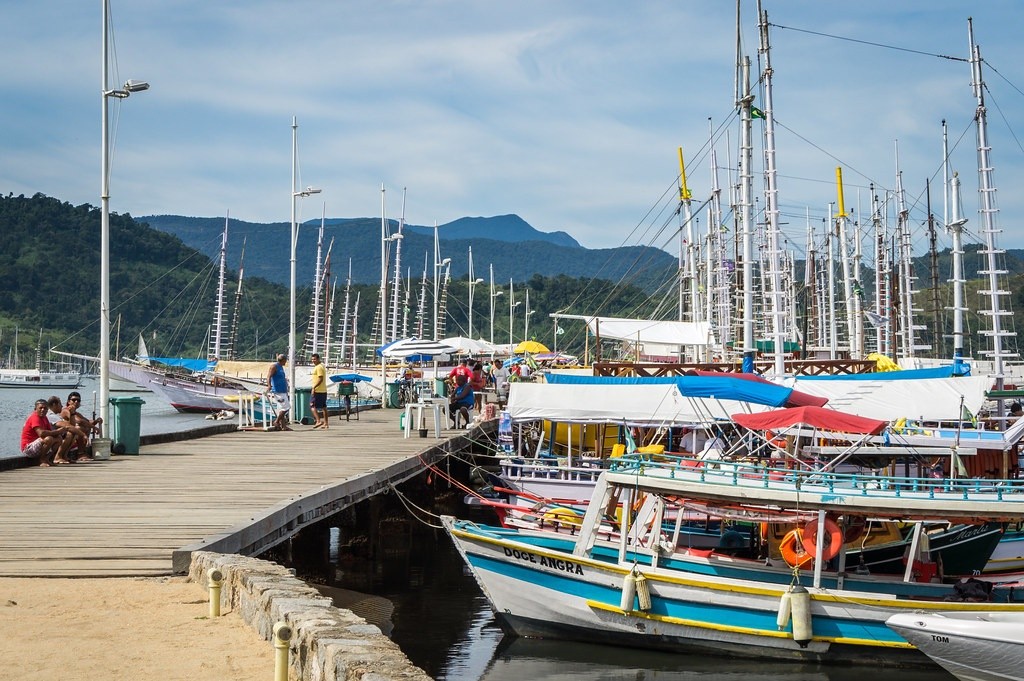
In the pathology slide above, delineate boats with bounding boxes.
[880,608,1024,681]
[400,337,1024,657]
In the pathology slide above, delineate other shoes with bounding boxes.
[75,453,94,461]
[461,425,466,429]
[451,422,460,429]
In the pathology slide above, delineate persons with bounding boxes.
[678,429,727,454]
[512,361,530,377]
[400,363,415,387]
[449,358,510,414]
[442,377,474,428]
[927,463,956,492]
[310,353,328,428]
[539,360,547,369]
[267,355,293,431]
[20,392,103,467]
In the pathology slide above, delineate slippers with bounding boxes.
[39,460,50,467]
[273,422,281,431]
[53,459,70,464]
[282,427,293,431]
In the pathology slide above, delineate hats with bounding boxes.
[1011,403,1022,412]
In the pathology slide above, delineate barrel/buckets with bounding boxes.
[91,438,112,461]
[419,429,428,437]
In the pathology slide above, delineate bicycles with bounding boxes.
[390,375,418,413]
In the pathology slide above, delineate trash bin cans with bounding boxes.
[386,381,404,408]
[109,397,146,455]
[295,387,315,425]
[435,377,449,398]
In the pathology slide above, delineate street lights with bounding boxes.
[284,115,324,423]
[524,289,536,342]
[85,0,147,461]
[488,262,504,342]
[468,245,484,339]
[433,219,452,344]
[509,277,522,348]
[379,181,404,413]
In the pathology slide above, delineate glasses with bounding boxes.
[70,398,81,404]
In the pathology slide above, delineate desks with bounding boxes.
[403,404,441,440]
[417,397,449,430]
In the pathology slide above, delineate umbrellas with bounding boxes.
[503,357,524,366]
[531,354,568,361]
[514,339,549,353]
[378,335,459,427]
[440,337,508,356]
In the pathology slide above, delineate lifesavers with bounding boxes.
[781,511,867,567]
